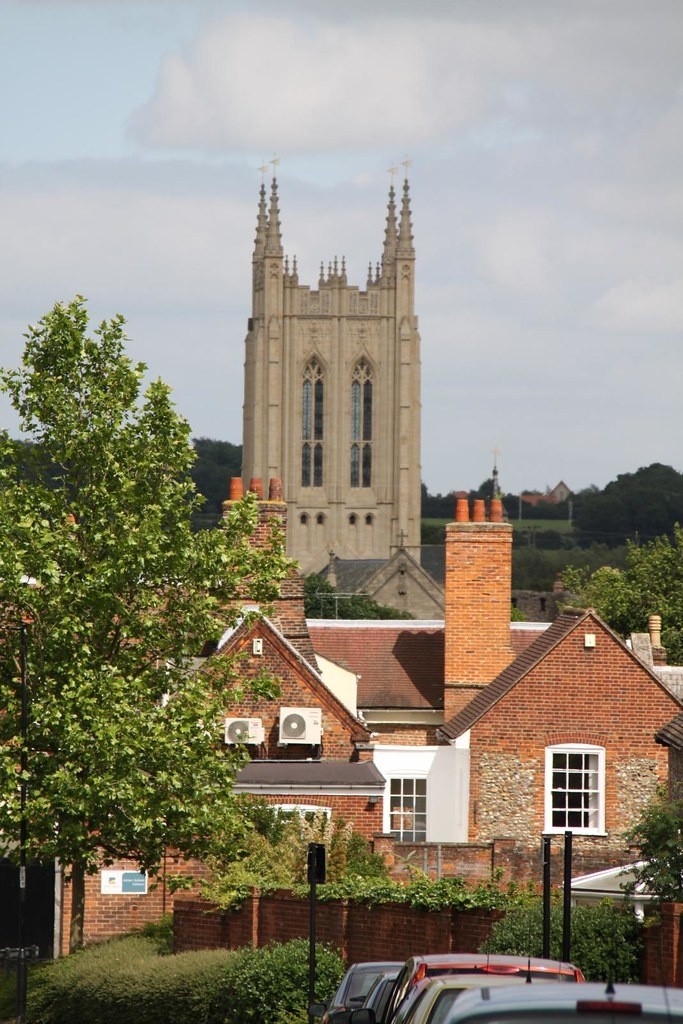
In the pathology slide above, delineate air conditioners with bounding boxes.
[279,706,321,745]
[224,718,262,745]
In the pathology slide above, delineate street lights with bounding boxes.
[17,576,37,1023]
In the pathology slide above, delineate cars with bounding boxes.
[307,951,683,1024]
